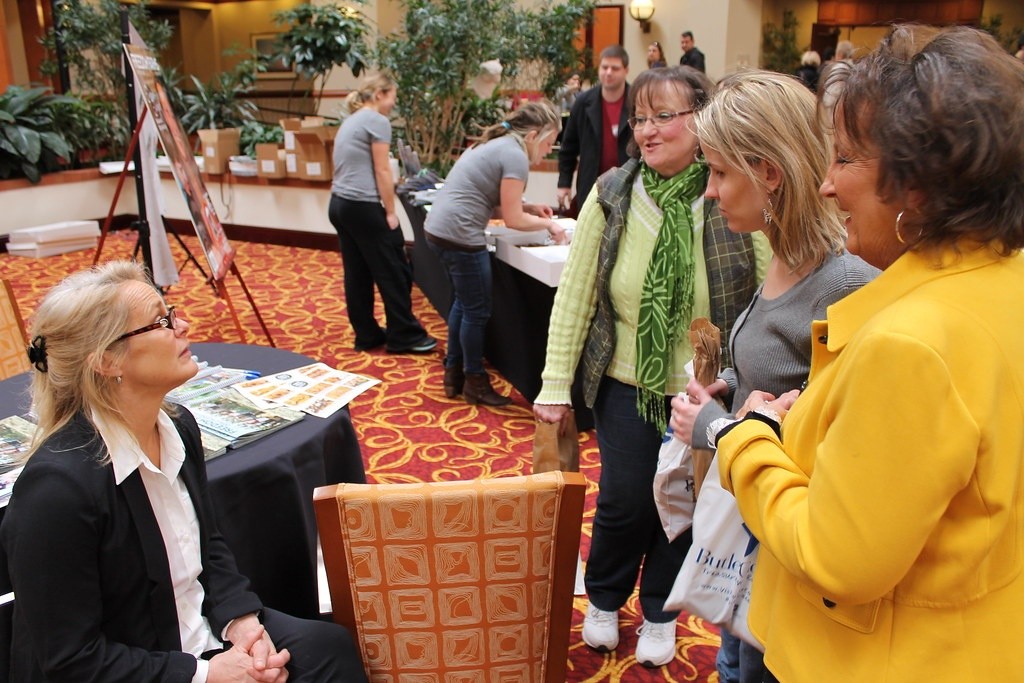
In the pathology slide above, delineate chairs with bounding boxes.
[316,472,587,683]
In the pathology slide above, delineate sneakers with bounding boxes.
[410,337,438,351]
[581,601,619,652]
[635,614,677,667]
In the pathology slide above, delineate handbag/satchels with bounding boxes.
[533,409,579,474]
[653,391,697,544]
[662,449,769,654]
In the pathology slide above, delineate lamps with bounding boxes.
[629,0,655,32]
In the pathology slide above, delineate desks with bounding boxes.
[0,344,365,618]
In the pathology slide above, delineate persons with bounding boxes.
[551,31,705,219]
[536,66,774,665]
[423,97,568,402]
[703,25,1023,683]
[328,75,439,354]
[669,68,883,683]
[154,75,213,254]
[0,258,367,683]
[795,41,855,93]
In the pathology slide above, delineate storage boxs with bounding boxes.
[196,116,337,181]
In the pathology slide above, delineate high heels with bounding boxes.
[463,375,512,406]
[443,373,463,398]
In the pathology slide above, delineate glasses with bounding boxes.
[105,306,176,350]
[627,110,694,131]
